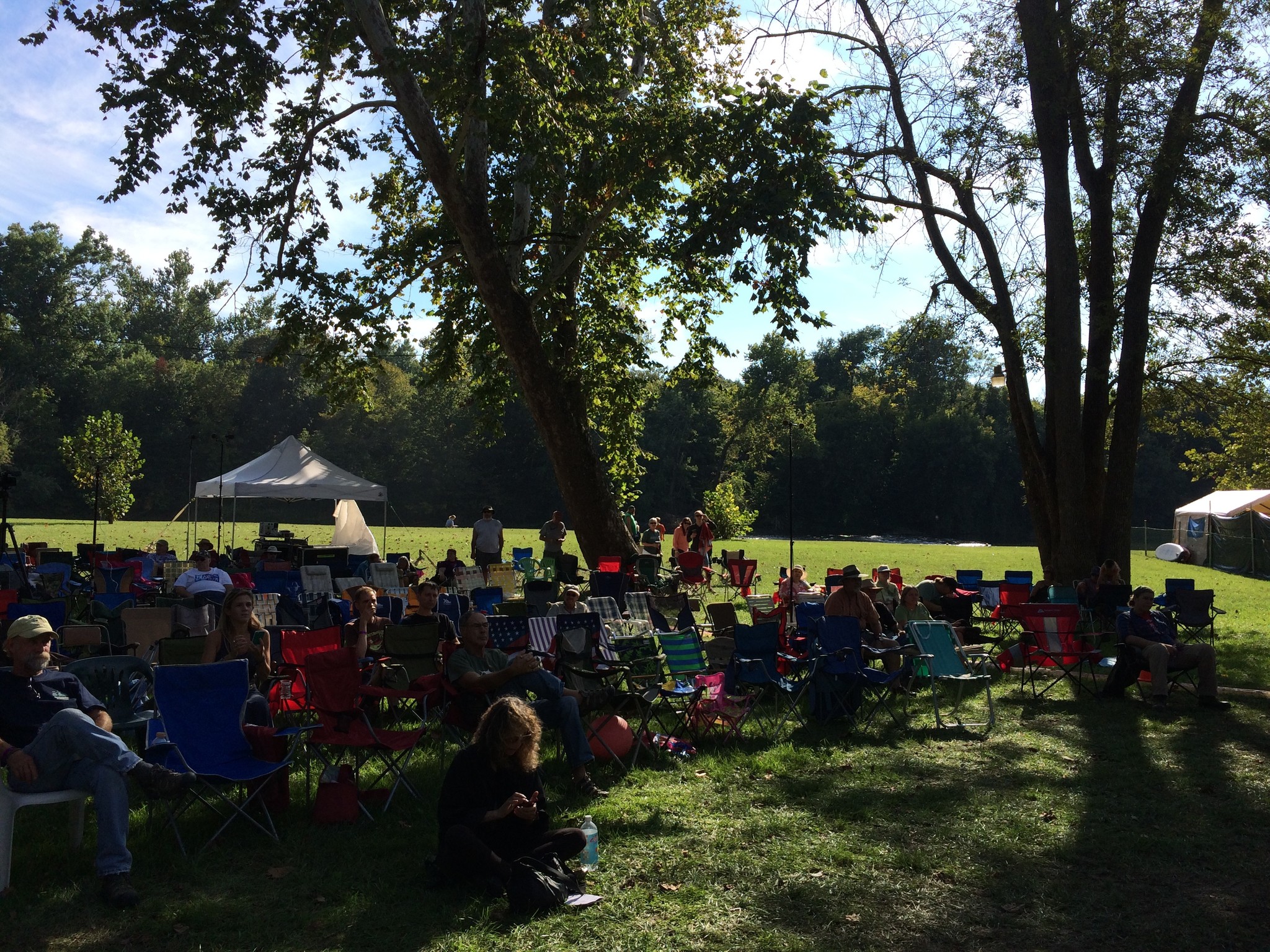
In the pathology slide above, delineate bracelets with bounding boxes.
[7,748,22,759]
[3,746,16,760]
[359,631,366,635]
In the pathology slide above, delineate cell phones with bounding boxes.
[253,631,265,645]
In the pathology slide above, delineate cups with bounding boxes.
[280,680,292,699]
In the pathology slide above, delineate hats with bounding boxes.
[786,565,807,580]
[263,546,282,554]
[682,517,692,525]
[1090,566,1100,574]
[7,614,60,641]
[196,550,211,558]
[196,539,213,550]
[944,577,958,592]
[877,565,892,574]
[155,540,168,546]
[860,579,884,594]
[480,505,495,514]
[564,584,580,597]
[838,565,867,585]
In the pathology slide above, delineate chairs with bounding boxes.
[0,540,1227,889]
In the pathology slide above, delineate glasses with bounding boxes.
[447,552,455,555]
[1092,574,1099,577]
[650,522,657,524]
[567,593,576,597]
[684,522,690,525]
[695,515,702,517]
[484,510,490,514]
[460,622,490,630]
[196,559,205,562]
[1043,570,1052,574]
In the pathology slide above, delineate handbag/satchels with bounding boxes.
[507,853,586,911]
[632,531,641,545]
[312,765,359,822]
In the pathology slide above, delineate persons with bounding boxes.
[255,546,285,572]
[624,505,717,595]
[470,505,504,585]
[781,559,1125,698]
[145,539,176,575]
[445,515,459,528]
[1116,586,1230,711]
[447,610,616,799]
[396,555,417,587]
[173,550,234,608]
[0,614,197,909]
[200,587,274,727]
[436,695,586,891]
[188,538,213,561]
[344,581,461,723]
[356,553,379,582]
[546,584,591,618]
[539,510,567,581]
[438,548,466,586]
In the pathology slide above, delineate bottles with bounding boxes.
[580,815,599,872]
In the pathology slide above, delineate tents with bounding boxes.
[1172,490,1270,566]
[194,435,387,561]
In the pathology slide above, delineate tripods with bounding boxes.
[0,488,33,600]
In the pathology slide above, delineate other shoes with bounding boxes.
[965,626,981,634]
[102,873,139,907]
[896,686,917,697]
[1153,696,1167,712]
[567,772,610,798]
[576,686,616,718]
[1198,695,1231,710]
[708,588,718,596]
[138,762,196,800]
